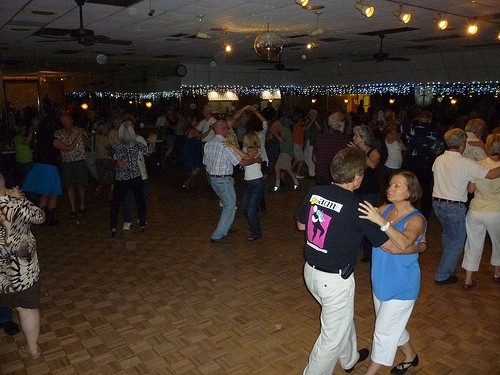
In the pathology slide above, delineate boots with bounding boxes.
[38,205,60,227]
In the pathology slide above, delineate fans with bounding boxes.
[351,35,411,62]
[257,53,301,71]
[36,0,132,46]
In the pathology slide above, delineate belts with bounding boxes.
[308,261,345,274]
[210,175,232,177]
[432,196,464,204]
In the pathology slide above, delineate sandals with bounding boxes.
[20,343,43,360]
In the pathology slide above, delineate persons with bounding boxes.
[295,148,426,375]
[0,88,500,242]
[459,133,499,290]
[0,186,32,334]
[355,170,426,375]
[429,127,500,285]
[231,132,267,241]
[0,171,47,357]
[202,117,263,245]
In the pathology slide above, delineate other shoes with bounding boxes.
[434,275,458,284]
[344,348,369,373]
[247,225,262,241]
[290,184,302,192]
[0,320,21,336]
[110,227,118,239]
[218,200,238,210]
[68,205,87,220]
[361,257,371,261]
[295,175,305,179]
[309,175,317,179]
[181,182,198,192]
[210,226,239,243]
[268,186,281,192]
[492,275,500,282]
[137,218,147,232]
[390,353,419,375]
[122,221,133,232]
[462,280,477,291]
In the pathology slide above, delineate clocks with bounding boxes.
[176,64,187,77]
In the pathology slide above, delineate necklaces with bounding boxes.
[389,205,413,224]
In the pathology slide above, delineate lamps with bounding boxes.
[432,10,448,30]
[355,1,376,18]
[307,36,320,49]
[220,31,232,52]
[295,0,310,7]
[392,3,411,24]
[465,17,479,36]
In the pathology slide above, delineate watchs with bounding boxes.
[381,222,390,231]
